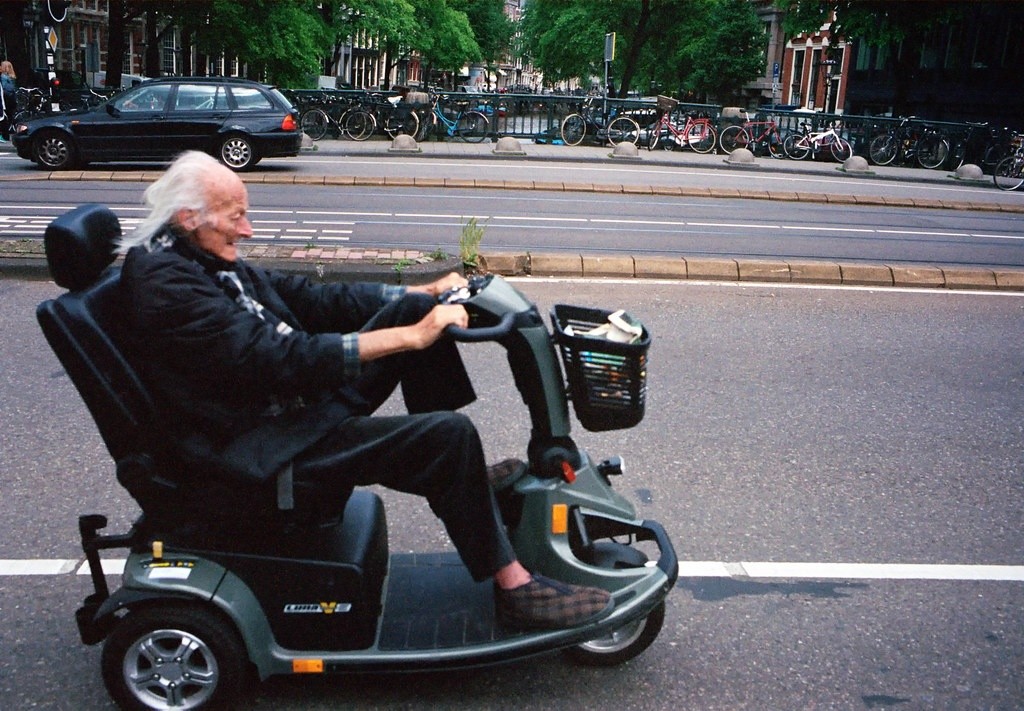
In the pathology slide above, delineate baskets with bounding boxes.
[550,304,652,432]
[658,95,678,112]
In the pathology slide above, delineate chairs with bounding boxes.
[35,201,388,603]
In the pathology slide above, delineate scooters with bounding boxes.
[34,206,681,711]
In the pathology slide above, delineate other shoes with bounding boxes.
[494,573,615,628]
[0,135,7,143]
[485,458,526,491]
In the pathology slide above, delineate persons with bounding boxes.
[116,152,615,630]
[124,90,166,111]
[474,72,482,92]
[0,61,17,143]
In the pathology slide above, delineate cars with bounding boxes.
[11,76,303,171]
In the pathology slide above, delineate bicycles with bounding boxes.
[9,81,1024,190]
